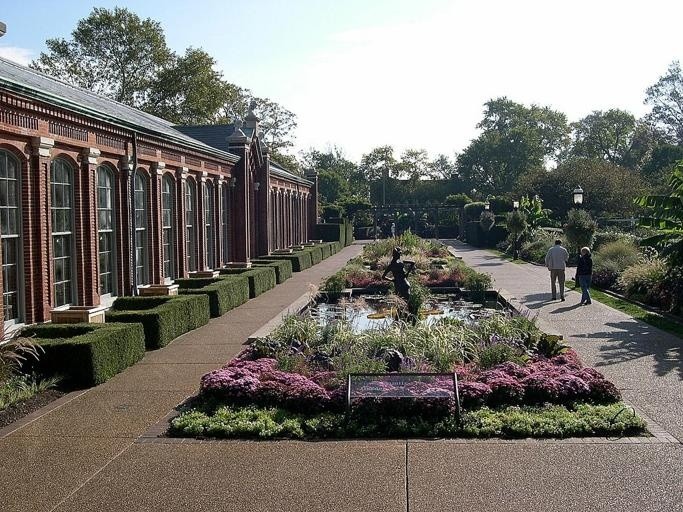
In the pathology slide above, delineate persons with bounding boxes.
[545,239,569,301]
[576,246,593,305]
[381,246,416,297]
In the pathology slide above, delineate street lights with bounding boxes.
[511,194,520,260]
[571,185,584,267]
[483,200,490,249]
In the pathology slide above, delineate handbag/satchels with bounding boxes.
[548,256,553,270]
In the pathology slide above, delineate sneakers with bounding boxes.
[586,301,591,304]
[552,298,556,300]
[562,299,565,301]
